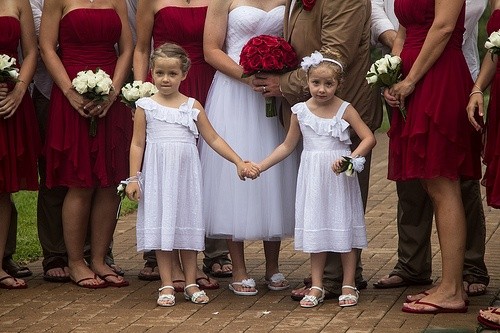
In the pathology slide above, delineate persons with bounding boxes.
[253,0,384,303]
[132,0,299,296]
[0,0,135,290]
[370,0,490,316]
[241,48,378,308]
[125,44,260,306]
[467,0,500,333]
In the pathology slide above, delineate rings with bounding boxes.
[14,103,16,107]
[97,105,102,109]
[263,87,266,92]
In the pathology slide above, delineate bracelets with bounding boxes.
[114,172,144,219]
[469,86,484,97]
[336,155,366,179]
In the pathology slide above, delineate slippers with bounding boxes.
[197,276,219,290]
[88,264,129,287]
[464,275,486,296]
[203,257,232,276]
[266,273,289,291]
[229,277,258,295]
[172,280,185,292]
[0,275,26,289]
[373,271,433,287]
[292,283,334,301]
[44,267,72,283]
[70,272,108,288]
[139,264,159,279]
[478,308,500,329]
[405,289,470,304]
[402,299,469,313]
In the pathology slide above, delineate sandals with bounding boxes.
[301,286,325,308]
[184,284,210,304]
[157,286,175,307]
[339,286,359,307]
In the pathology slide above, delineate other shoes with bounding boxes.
[2,257,31,276]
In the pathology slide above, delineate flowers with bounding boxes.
[119,80,158,109]
[365,53,407,119]
[484,28,500,62]
[70,68,115,135]
[0,53,25,85]
[239,34,300,117]
